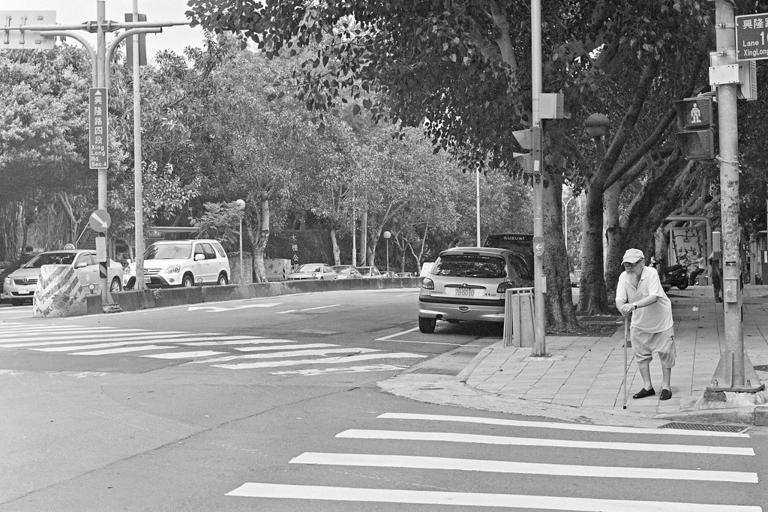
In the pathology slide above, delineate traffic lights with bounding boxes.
[509,129,533,173]
[671,97,714,161]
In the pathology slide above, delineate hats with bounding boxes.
[621,248,644,266]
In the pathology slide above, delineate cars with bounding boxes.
[418,233,534,333]
[287,263,413,279]
[0,239,231,305]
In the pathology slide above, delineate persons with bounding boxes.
[690,252,705,286]
[23,245,35,263]
[615,248,677,399]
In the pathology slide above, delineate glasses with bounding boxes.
[624,262,638,267]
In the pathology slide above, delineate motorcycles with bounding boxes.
[650,256,688,290]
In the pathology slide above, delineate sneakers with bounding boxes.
[660,389,672,400]
[633,387,655,399]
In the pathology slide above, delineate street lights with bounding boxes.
[383,231,391,276]
[237,199,245,275]
